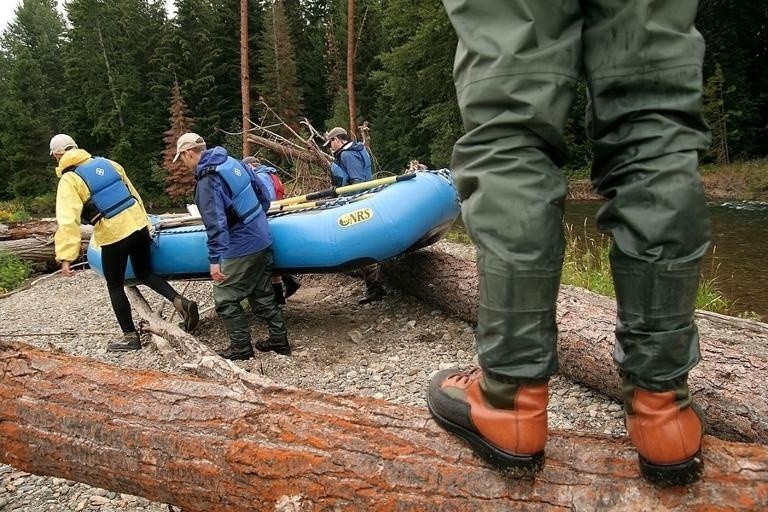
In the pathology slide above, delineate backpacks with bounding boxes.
[269,172,284,200]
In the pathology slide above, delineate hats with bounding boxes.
[323,127,347,147]
[172,133,206,163]
[242,156,258,164]
[49,134,78,156]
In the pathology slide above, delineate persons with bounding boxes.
[49,134,199,352]
[172,132,291,360]
[323,126,387,304]
[427,0,713,486]
[242,156,301,304]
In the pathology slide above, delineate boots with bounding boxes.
[272,282,286,305]
[282,273,299,298]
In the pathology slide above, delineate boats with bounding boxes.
[86,171,460,279]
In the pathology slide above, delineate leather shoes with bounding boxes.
[359,298,382,304]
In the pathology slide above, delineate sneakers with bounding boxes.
[621,381,705,485]
[427,370,548,475]
[174,296,199,330]
[255,335,291,355]
[108,332,142,352]
[215,342,254,360]
